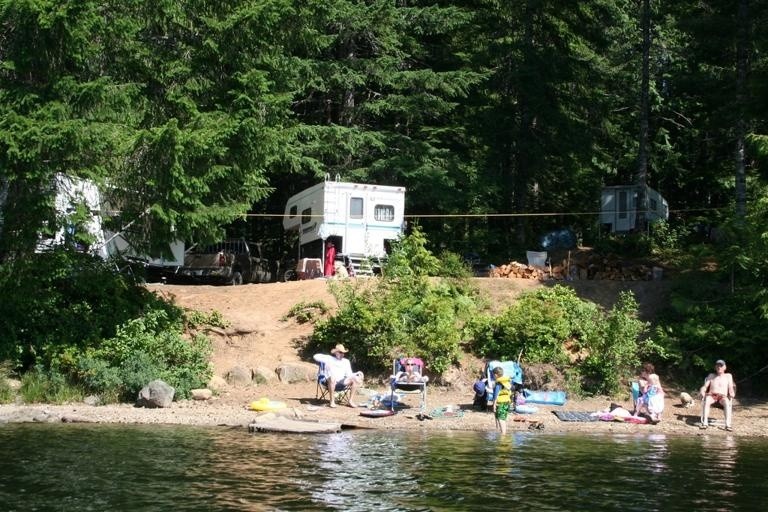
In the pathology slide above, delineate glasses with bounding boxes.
[407,363,413,366]
[338,351,345,354]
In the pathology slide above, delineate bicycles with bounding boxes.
[284,269,296,283]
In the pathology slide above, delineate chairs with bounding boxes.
[317,351,354,405]
[389,356,427,413]
[700,379,735,429]
[486,359,522,413]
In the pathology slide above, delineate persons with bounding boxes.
[396,359,429,382]
[636,360,665,419]
[323,343,363,408]
[492,367,512,435]
[700,360,736,430]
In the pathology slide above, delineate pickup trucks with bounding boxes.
[185,237,279,285]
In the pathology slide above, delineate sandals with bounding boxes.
[725,425,733,432]
[700,423,708,429]
[514,416,541,422]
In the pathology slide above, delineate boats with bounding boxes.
[248,413,342,433]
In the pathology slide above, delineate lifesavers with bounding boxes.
[251,397,286,410]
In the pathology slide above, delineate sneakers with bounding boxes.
[527,423,545,431]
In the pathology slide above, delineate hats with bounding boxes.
[473,381,485,397]
[715,360,725,366]
[331,343,349,354]
